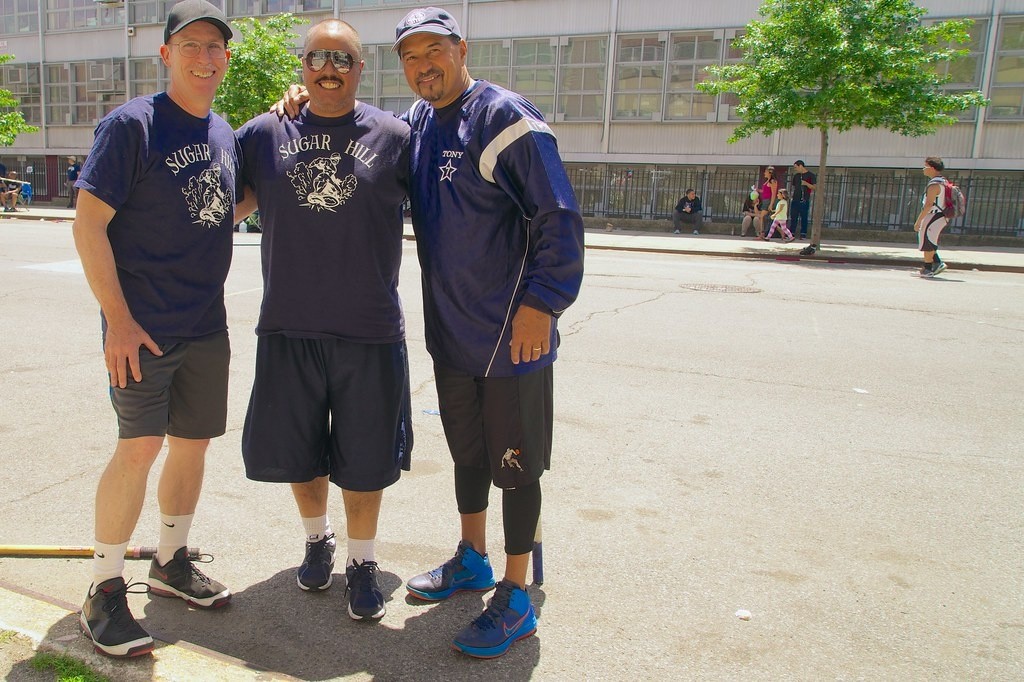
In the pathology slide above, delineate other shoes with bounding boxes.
[674,230,680,233]
[693,231,698,235]
[67,204,73,208]
[786,237,795,242]
[800,233,807,239]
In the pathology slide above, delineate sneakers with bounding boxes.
[406,540,497,601]
[451,578,536,659]
[78,577,155,659]
[147,547,232,609]
[296,532,336,590]
[933,263,947,275]
[344,557,384,620]
[911,267,934,277]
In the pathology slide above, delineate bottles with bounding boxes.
[239,221,248,233]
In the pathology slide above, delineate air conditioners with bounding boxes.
[8,68,37,84]
[90,64,120,81]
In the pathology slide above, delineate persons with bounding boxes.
[754,167,787,240]
[741,195,764,237]
[65,156,81,208]
[764,189,795,243]
[268,6,585,659]
[910,156,951,278]
[790,160,816,240]
[673,189,704,235]
[0,162,7,192]
[0,171,22,212]
[236,19,413,622]
[71,0,275,659]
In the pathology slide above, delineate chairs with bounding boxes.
[6,183,33,213]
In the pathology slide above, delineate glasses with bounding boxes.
[302,49,359,74]
[169,41,230,59]
[922,166,933,169]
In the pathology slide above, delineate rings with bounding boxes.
[534,347,541,351]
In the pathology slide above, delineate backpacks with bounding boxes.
[925,182,966,218]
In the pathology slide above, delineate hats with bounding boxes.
[390,7,462,53]
[750,191,759,200]
[67,156,76,162]
[164,0,233,45]
